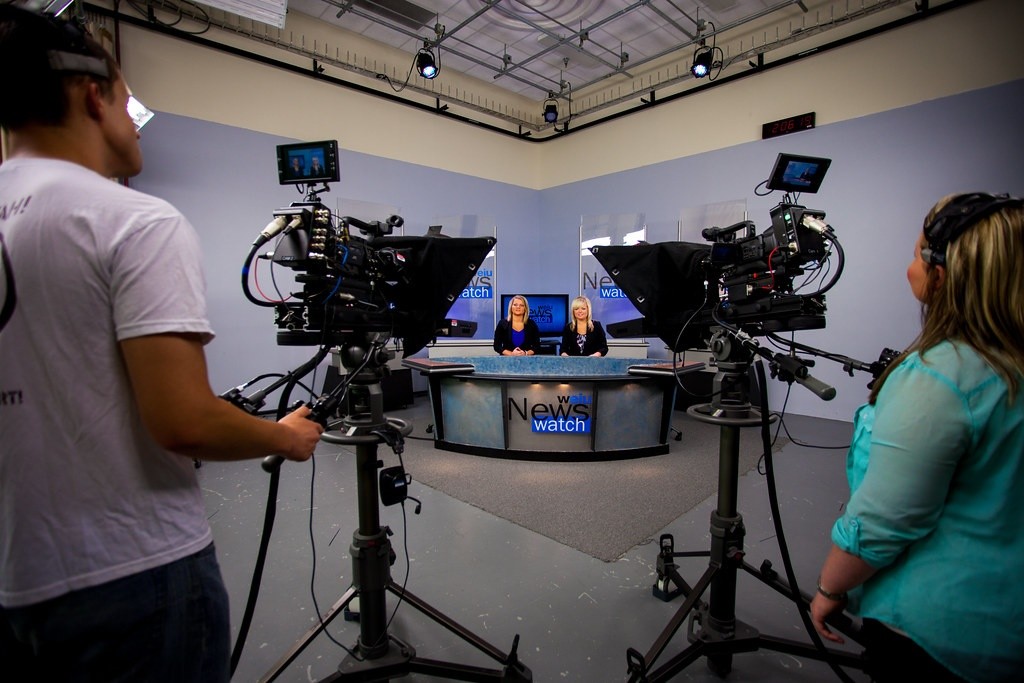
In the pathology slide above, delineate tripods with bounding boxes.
[622,326,873,683]
[217,327,532,683]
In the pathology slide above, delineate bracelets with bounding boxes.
[524,350,528,356]
[817,574,845,602]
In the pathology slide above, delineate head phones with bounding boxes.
[921,192,1024,267]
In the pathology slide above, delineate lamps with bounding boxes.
[690,45,714,78]
[542,98,560,122]
[414,47,438,79]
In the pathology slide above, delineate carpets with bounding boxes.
[321,392,794,562]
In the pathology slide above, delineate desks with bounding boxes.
[427,339,501,356]
[424,356,680,462]
[328,346,413,410]
[604,339,651,359]
[664,344,720,412]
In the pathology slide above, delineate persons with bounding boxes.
[309,157,324,176]
[559,296,608,357]
[290,158,304,178]
[493,295,540,356]
[0,0,326,683]
[808,193,1024,683]
[799,167,810,182]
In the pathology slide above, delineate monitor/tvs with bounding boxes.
[501,293,569,337]
